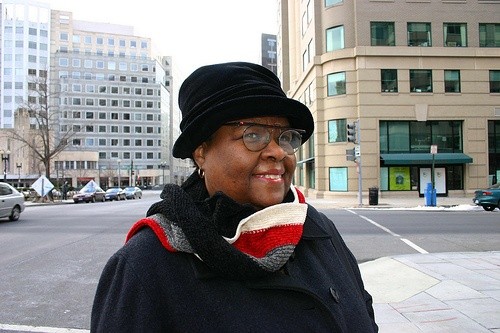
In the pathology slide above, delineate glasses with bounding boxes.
[222,121,306,156]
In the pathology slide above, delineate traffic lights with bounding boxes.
[132,170,135,175]
[346,121,356,143]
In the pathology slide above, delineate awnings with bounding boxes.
[380,152,473,164]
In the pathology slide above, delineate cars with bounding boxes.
[0,182,24,222]
[123,186,143,199]
[473,183,500,211]
[72,186,107,203]
[105,188,128,201]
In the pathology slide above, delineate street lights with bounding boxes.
[0,147,12,183]
[117,159,122,189]
[160,161,168,188]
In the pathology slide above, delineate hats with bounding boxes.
[172,62,315,160]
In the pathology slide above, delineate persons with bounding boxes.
[61,181,68,200]
[90,61,379,333]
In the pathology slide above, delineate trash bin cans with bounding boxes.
[368,187,379,206]
